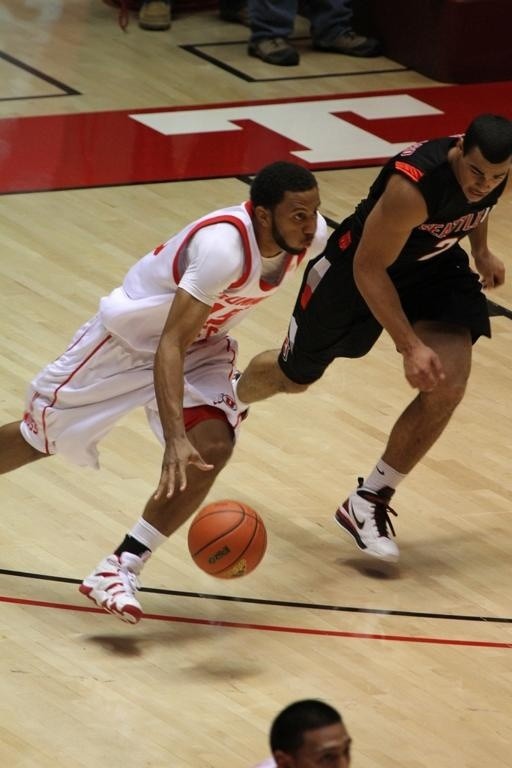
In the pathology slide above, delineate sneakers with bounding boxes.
[79,551,144,624]
[334,476,399,563]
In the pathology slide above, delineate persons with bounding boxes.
[137,0,313,40]
[0,160,331,624]
[249,0,382,65]
[251,699,354,768]
[235,113,511,564]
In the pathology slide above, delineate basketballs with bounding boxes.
[189,500,266,577]
